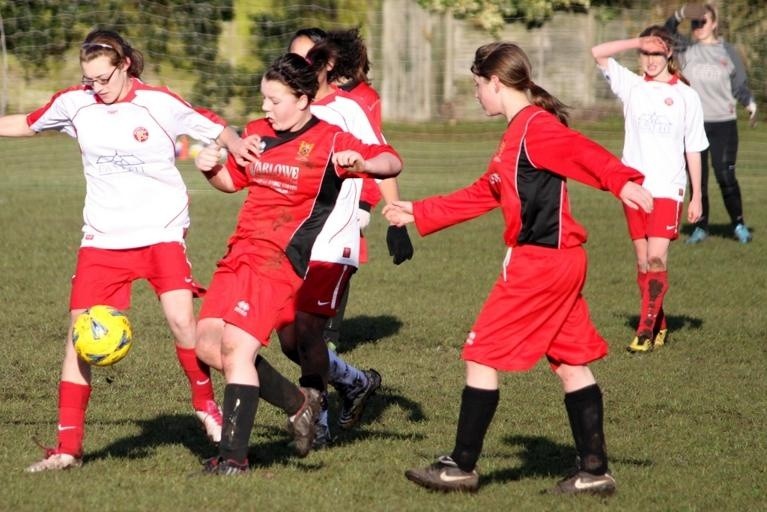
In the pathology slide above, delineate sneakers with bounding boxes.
[653,329,669,347]
[734,224,752,244]
[24,450,83,472]
[195,368,383,477]
[540,469,617,498]
[686,227,709,245]
[626,336,653,354]
[406,456,480,492]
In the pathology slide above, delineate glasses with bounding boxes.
[82,65,120,86]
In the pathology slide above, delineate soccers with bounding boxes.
[72,304,130,364]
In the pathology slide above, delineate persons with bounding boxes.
[1,28,261,474]
[381,42,654,497]
[194,17,403,476]
[276,24,414,449]
[665,3,758,244]
[591,25,711,353]
[325,30,382,348]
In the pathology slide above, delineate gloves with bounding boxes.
[386,225,413,265]
